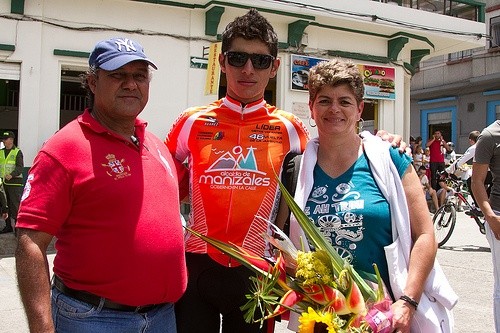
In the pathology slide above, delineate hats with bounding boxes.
[89,38,158,72]
[0,131,15,139]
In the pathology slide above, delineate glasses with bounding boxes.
[223,50,275,70]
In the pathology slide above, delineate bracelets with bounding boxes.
[400,296,418,311]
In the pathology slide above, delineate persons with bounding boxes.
[410,136,432,200]
[0,131,24,235]
[471,120,500,333]
[441,130,493,217]
[164,9,408,333]
[274,59,438,333]
[443,142,456,166]
[426,127,450,214]
[14,36,188,333]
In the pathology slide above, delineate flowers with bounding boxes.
[181,149,396,333]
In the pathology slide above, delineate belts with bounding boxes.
[52,275,169,313]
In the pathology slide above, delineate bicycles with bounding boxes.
[432,175,487,246]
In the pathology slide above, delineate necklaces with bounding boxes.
[130,135,138,147]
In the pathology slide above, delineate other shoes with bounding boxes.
[464,208,484,217]
[14,227,17,237]
[0,225,14,234]
